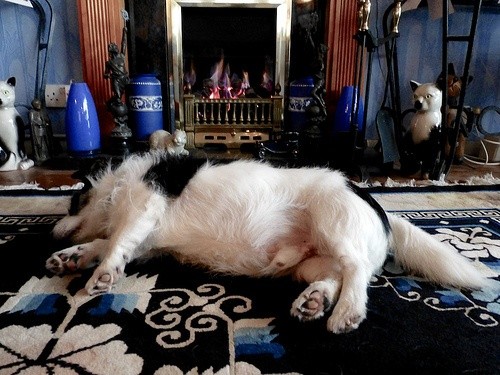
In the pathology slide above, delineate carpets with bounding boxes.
[0,172,500,375]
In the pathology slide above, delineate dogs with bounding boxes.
[48,147,494,334]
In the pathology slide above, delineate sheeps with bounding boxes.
[149,129,191,156]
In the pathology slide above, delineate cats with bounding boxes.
[0,76,35,172]
[392,62,476,187]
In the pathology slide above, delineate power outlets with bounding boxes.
[45,84,71,107]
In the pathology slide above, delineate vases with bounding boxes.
[131,73,163,138]
[335,85,364,131]
[288,78,314,125]
[65,82,101,158]
